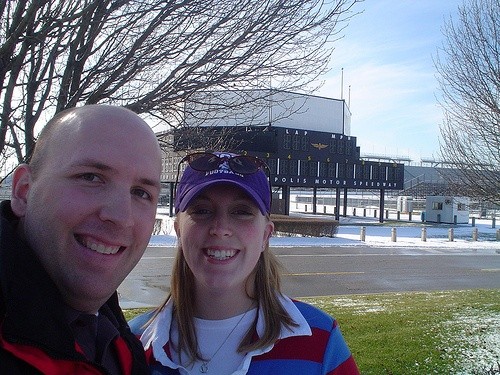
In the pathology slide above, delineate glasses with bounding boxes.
[174,152,272,217]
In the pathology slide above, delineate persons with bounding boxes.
[0,105,183,375]
[127,150,362,375]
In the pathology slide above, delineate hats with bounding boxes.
[175,152,271,216]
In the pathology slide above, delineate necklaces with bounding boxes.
[196,301,256,375]
